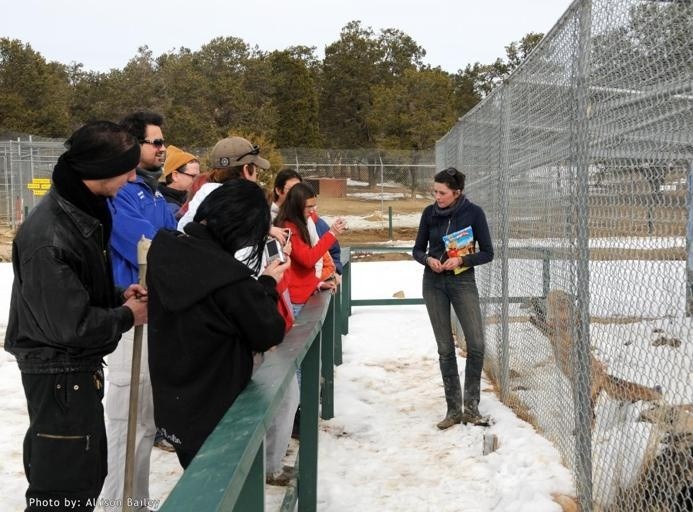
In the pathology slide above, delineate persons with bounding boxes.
[0,115,149,512]
[95,111,347,512]
[412,167,494,429]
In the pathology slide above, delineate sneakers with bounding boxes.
[265,463,298,485]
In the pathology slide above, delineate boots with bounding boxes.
[437,375,463,430]
[463,374,490,426]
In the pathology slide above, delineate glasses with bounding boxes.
[236,144,260,162]
[135,138,166,146]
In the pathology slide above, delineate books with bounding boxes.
[443,225,475,277]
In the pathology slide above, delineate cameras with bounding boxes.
[264,238,287,266]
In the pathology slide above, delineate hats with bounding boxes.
[208,135,271,171]
[164,144,197,177]
